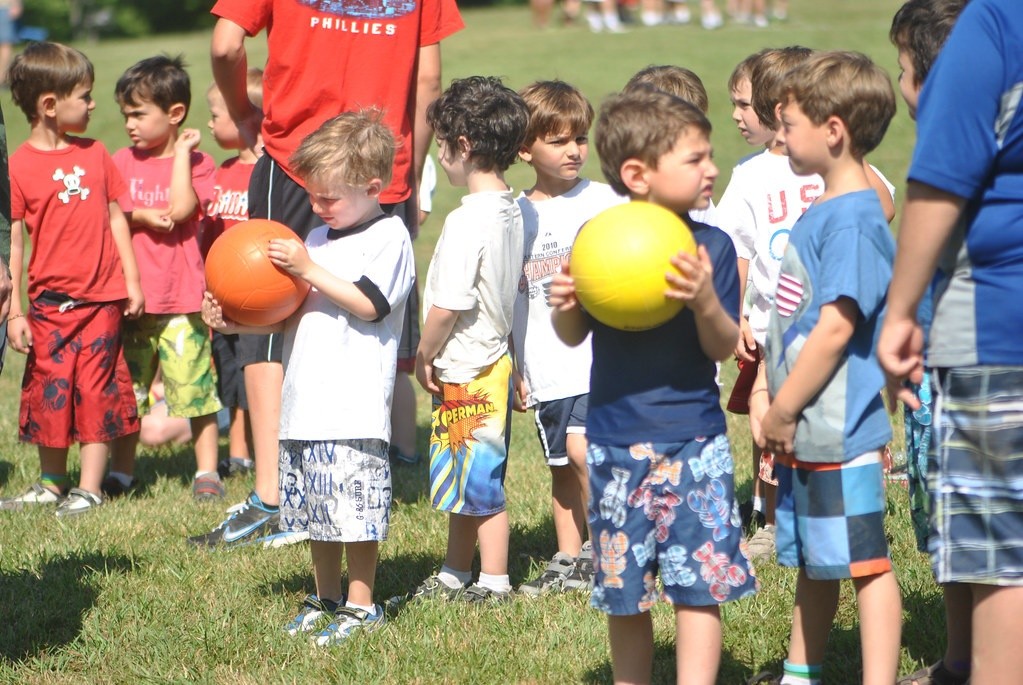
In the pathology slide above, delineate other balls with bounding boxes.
[567,201,700,333]
[204,218,313,327]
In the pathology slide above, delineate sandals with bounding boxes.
[896,659,965,685]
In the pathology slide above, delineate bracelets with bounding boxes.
[8,313,23,321]
[751,388,768,397]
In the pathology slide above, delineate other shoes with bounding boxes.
[739,499,766,533]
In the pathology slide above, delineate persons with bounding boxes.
[874,0,1023,685]
[0,0,22,86]
[186,0,466,554]
[508,81,630,598]
[549,43,903,685]
[0,39,145,516]
[529,0,789,33]
[389,76,532,608]
[101,55,225,502]
[888,0,973,685]
[201,68,265,479]
[201,110,416,647]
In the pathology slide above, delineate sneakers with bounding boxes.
[0,473,71,507]
[217,457,254,480]
[562,541,595,590]
[102,475,136,499]
[188,490,310,552]
[307,604,385,646]
[459,583,515,608]
[193,473,226,502]
[747,524,776,563]
[518,552,577,595]
[287,593,344,636]
[54,487,103,515]
[390,575,475,607]
